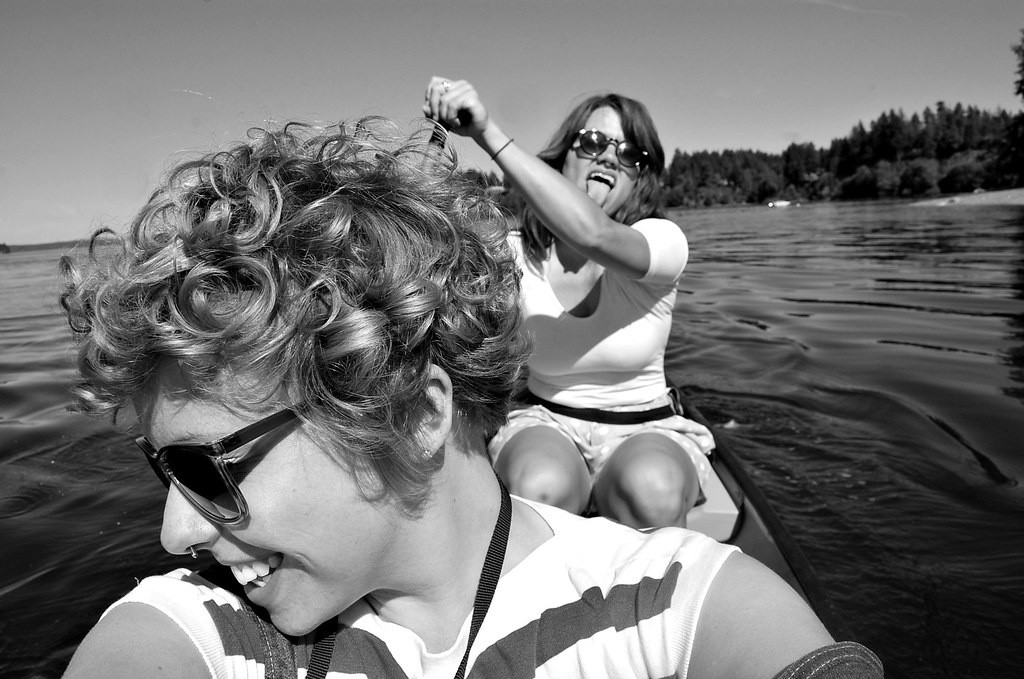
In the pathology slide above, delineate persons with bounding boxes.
[59,114,886,679]
[423,74,716,533]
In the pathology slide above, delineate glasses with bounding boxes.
[580,129,649,167]
[134,397,328,526]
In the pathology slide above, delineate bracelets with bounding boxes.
[492,137,514,160]
